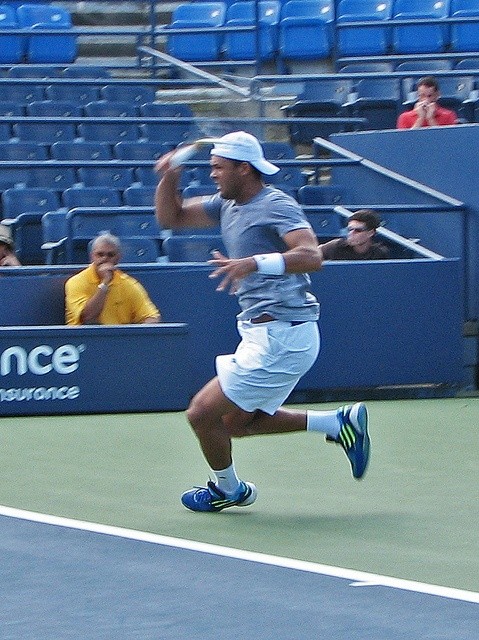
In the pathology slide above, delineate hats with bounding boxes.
[209,130,281,176]
[0,225,15,252]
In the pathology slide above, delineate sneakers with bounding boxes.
[325,402,370,479]
[180,475,257,512]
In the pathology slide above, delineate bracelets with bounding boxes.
[98,283,108,292]
[252,253,286,275]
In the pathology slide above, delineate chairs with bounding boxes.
[335,1,479,132]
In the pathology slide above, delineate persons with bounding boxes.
[154,127,373,514]
[0,223,22,267]
[317,210,392,261]
[63,231,161,325]
[396,76,457,130]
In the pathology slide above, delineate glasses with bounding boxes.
[346,226,374,233]
